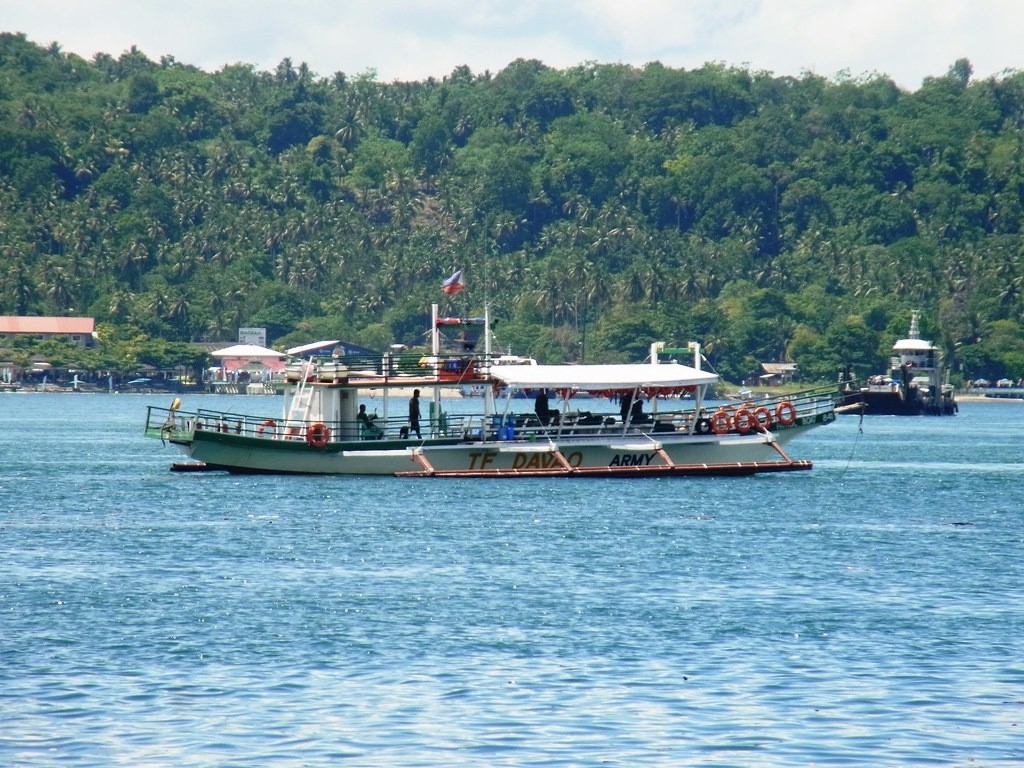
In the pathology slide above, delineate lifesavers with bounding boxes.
[711,411,731,433]
[256,421,277,439]
[776,402,796,426]
[733,408,753,433]
[695,418,713,435]
[753,406,772,433]
[305,423,330,447]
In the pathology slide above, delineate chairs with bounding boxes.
[356,420,380,440]
[429,401,447,437]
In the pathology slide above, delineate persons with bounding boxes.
[409,389,422,438]
[357,404,383,440]
[534,388,559,434]
[620,392,643,434]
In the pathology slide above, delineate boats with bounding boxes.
[836,307,959,416]
[142,301,870,479]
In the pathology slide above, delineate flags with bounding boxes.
[444,269,465,295]
